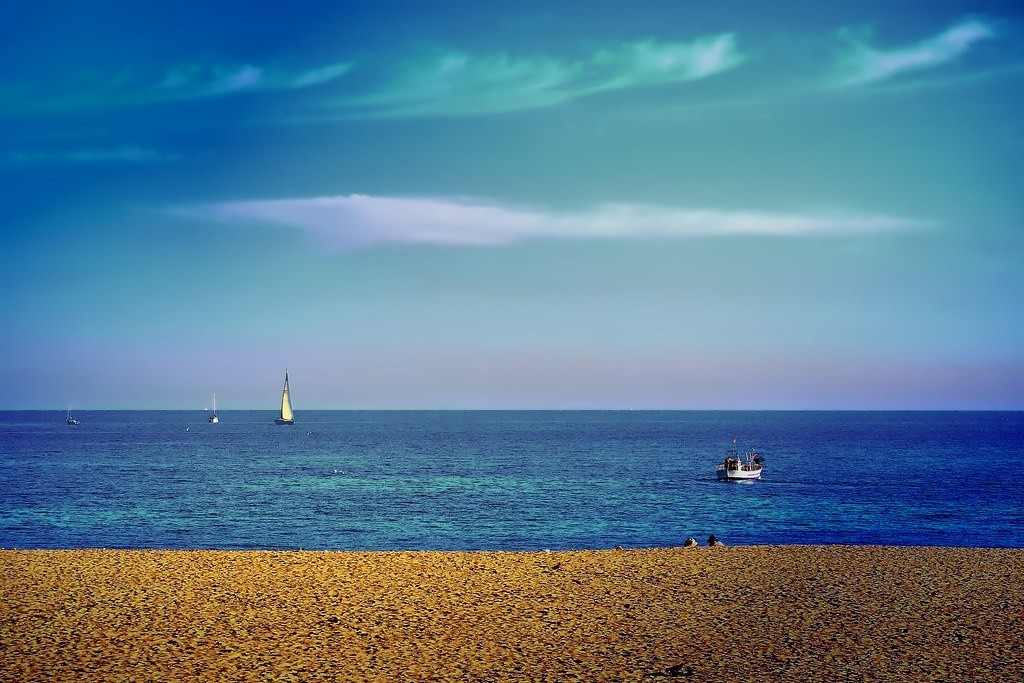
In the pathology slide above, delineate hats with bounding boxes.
[707,535,717,542]
[684,538,697,546]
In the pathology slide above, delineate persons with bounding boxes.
[684,537,698,547]
[706,534,725,547]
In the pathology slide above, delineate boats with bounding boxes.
[717,450,763,483]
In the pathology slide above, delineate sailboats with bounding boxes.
[273,367,296,426]
[209,395,220,424]
[65,396,79,425]
